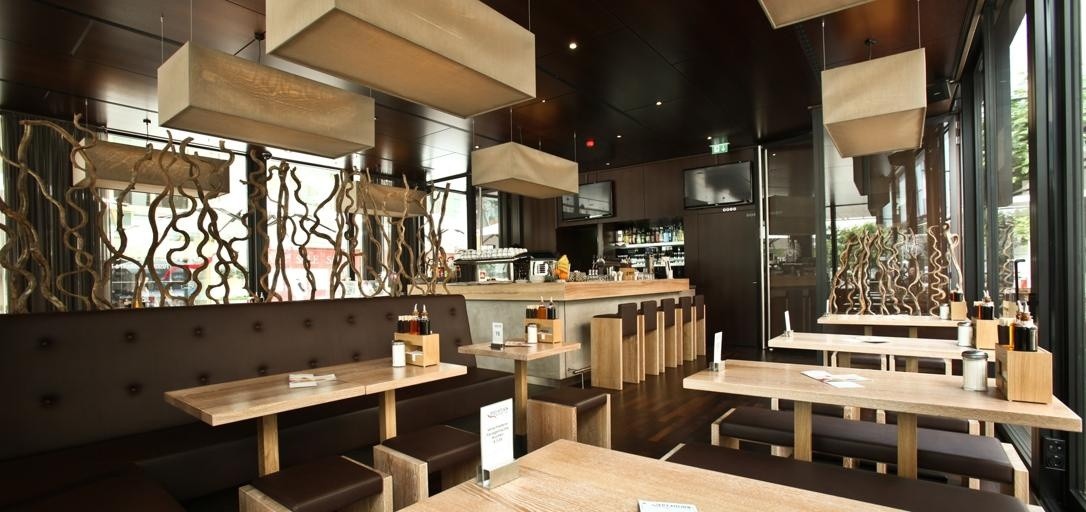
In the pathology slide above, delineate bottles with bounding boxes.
[956,321,974,347]
[960,349,990,393]
[939,302,950,320]
[390,338,407,368]
[420,304,430,335]
[547,296,556,320]
[1013,300,1039,352]
[538,296,547,319]
[526,322,539,344]
[618,224,685,267]
[409,304,420,335]
[980,290,995,320]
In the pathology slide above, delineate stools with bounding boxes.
[589,293,708,389]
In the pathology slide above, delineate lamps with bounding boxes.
[761,0,930,162]
[471,108,578,198]
[340,150,426,218]
[71,99,230,199]
[265,2,536,119]
[156,1,374,158]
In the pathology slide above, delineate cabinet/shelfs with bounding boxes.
[598,217,684,269]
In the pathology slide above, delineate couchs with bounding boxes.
[0,293,513,509]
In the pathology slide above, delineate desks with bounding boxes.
[163,313,1083,512]
